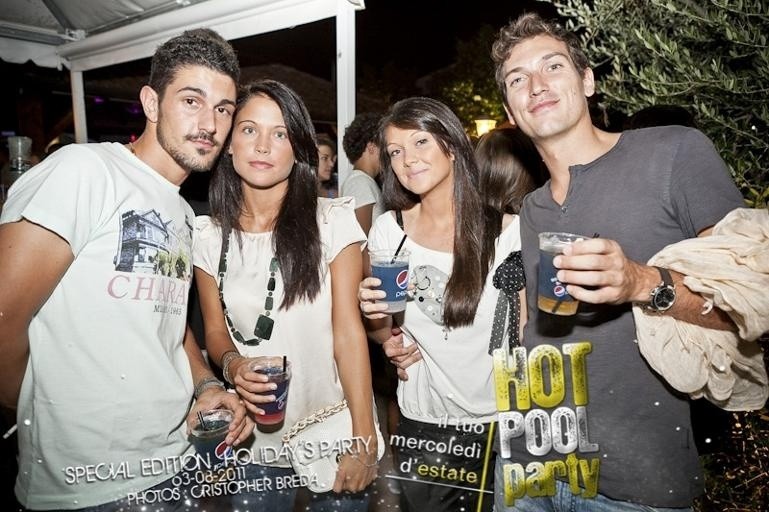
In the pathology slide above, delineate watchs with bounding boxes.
[636,265,674,312]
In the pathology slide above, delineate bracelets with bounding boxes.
[220,351,243,387]
[192,377,228,396]
[348,447,378,468]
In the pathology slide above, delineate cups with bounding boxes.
[247,355,292,426]
[188,410,237,482]
[535,232,594,318]
[365,249,413,314]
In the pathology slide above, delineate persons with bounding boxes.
[341,113,386,238]
[357,97,527,511]
[189,77,386,511]
[317,134,338,200]
[469,129,551,212]
[0,28,257,511]
[488,12,767,512]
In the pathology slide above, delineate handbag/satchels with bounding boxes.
[393,424,497,486]
[282,400,386,496]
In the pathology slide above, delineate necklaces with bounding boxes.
[214,202,292,351]
